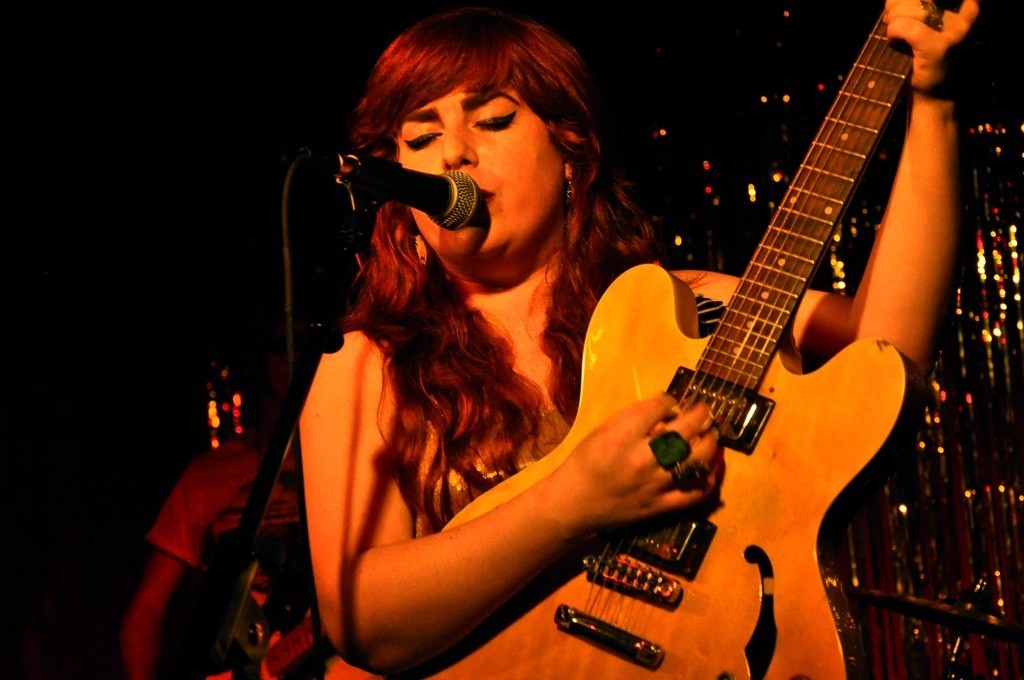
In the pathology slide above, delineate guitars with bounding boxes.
[408,12,930,680]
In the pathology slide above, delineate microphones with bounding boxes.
[298,148,486,232]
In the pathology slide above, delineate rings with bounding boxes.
[648,430,690,467]
[671,459,710,492]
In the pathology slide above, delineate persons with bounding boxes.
[296,0,980,674]
[119,318,326,680]
